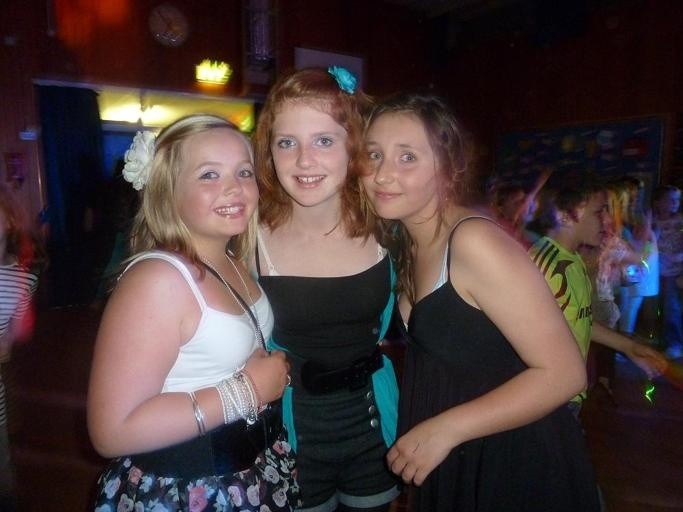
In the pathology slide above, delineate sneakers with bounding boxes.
[593,383,619,417]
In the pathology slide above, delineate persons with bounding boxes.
[236,64,398,511]
[2,152,683,414]
[0,183,41,511]
[355,92,592,511]
[75,109,301,511]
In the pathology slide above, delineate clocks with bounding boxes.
[142,4,198,48]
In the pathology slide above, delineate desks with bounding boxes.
[565,312,682,511]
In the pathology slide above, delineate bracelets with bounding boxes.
[214,369,267,427]
[184,386,209,441]
